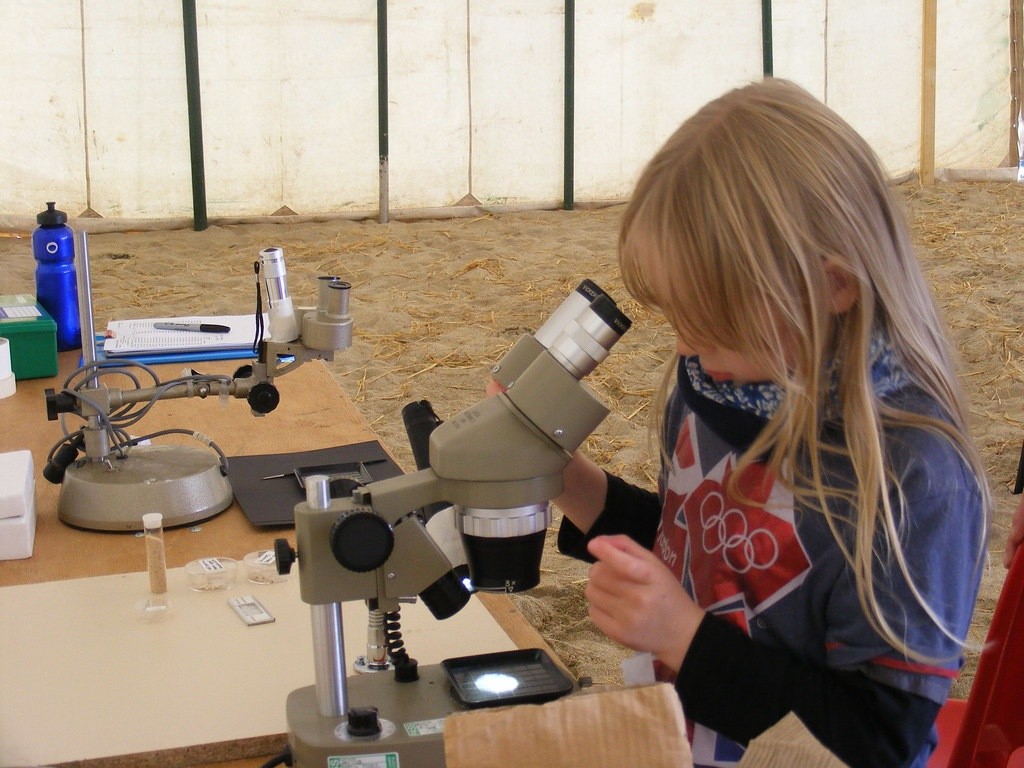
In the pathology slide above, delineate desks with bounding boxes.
[0,345,578,768]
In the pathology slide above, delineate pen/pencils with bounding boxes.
[153,322,230,333]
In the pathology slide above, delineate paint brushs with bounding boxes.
[257,457,386,481]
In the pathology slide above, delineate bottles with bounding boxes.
[33,202,82,352]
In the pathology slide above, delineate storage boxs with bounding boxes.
[0,294,59,380]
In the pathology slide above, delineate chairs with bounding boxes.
[923,541,1024,768]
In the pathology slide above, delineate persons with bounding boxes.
[486,79,1000,768]
